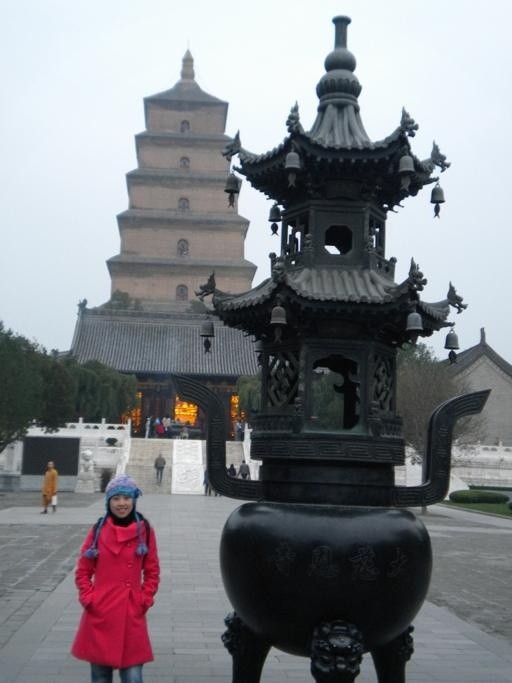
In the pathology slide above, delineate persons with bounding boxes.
[143,413,173,439]
[155,454,165,483]
[202,470,212,496]
[214,492,222,497]
[227,464,236,477]
[40,461,58,514]
[71,474,161,683]
[236,419,243,441]
[237,460,250,479]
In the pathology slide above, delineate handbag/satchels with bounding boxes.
[51,495,58,506]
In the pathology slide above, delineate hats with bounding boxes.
[104,475,142,503]
